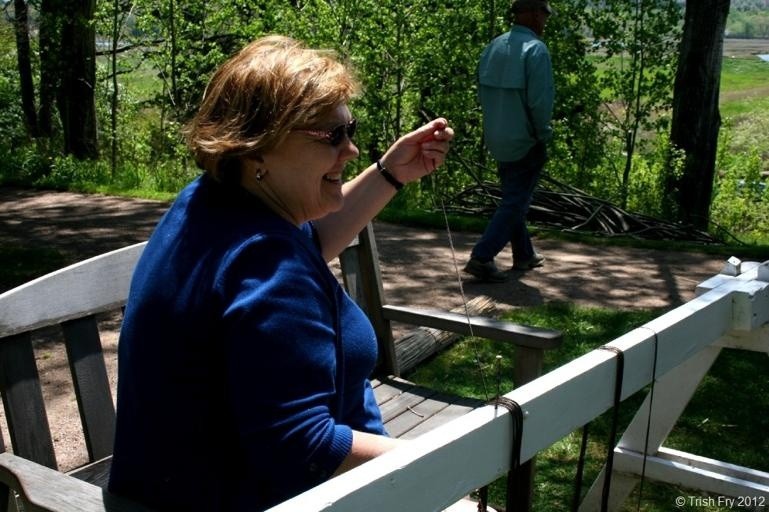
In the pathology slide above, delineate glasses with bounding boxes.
[289,117,358,147]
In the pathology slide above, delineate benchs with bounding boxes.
[0,219,561,512]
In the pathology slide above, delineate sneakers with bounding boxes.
[513,252,546,271]
[463,259,509,283]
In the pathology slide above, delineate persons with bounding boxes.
[456,5,559,280]
[104,27,501,512]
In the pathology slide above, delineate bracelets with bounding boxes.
[376,160,405,192]
